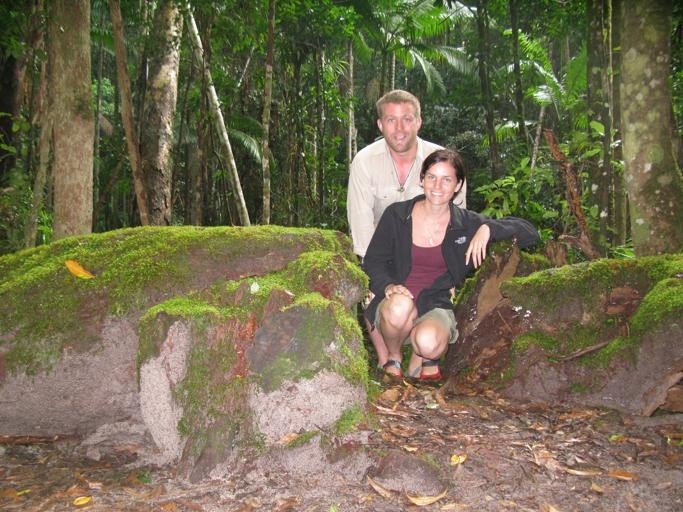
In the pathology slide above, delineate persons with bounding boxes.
[343,88,468,387]
[360,146,539,387]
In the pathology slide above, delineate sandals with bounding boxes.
[369,359,444,386]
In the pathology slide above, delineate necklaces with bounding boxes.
[420,204,448,246]
[390,151,415,193]
